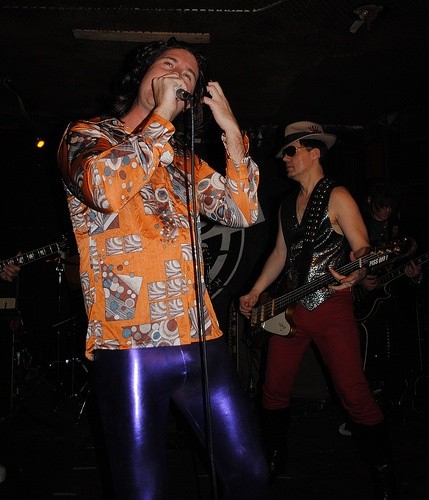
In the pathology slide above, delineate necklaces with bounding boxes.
[297,192,305,224]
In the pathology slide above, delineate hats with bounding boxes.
[277,120,337,157]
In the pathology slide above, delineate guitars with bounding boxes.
[224,243,410,369]
[0,233,71,277]
[351,249,429,324]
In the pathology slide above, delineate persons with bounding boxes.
[352,186,429,407]
[238,119,403,500]
[54,35,264,500]
[0,235,96,430]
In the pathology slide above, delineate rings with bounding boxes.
[406,273,409,275]
[408,271,411,274]
[371,288,374,290]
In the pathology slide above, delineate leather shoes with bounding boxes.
[354,421,400,500]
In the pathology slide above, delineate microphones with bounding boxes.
[176,88,193,101]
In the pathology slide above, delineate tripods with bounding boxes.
[0,256,96,427]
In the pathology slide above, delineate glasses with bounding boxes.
[283,145,312,157]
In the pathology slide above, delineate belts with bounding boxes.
[338,287,352,291]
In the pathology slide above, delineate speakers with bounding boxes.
[230,291,328,404]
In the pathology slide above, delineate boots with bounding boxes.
[263,407,290,472]
